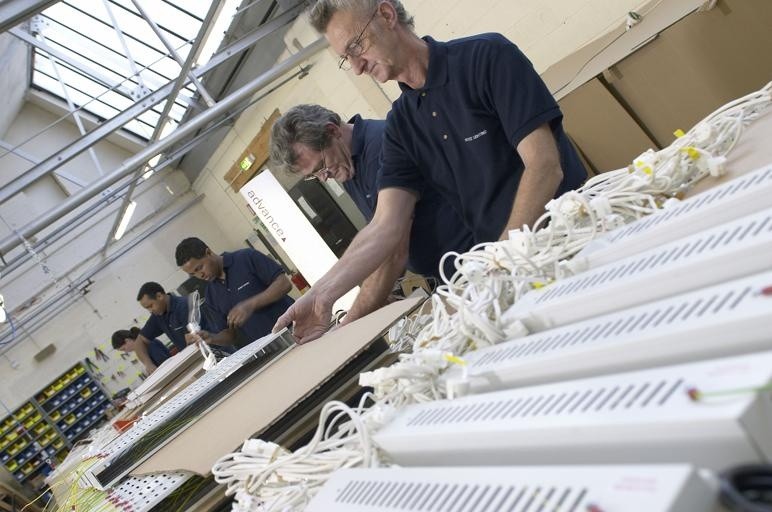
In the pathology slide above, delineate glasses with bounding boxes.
[339,5,380,72]
[306,149,327,183]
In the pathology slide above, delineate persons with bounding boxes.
[112,282,236,376]
[176,238,295,348]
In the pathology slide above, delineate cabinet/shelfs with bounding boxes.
[1,360,117,493]
